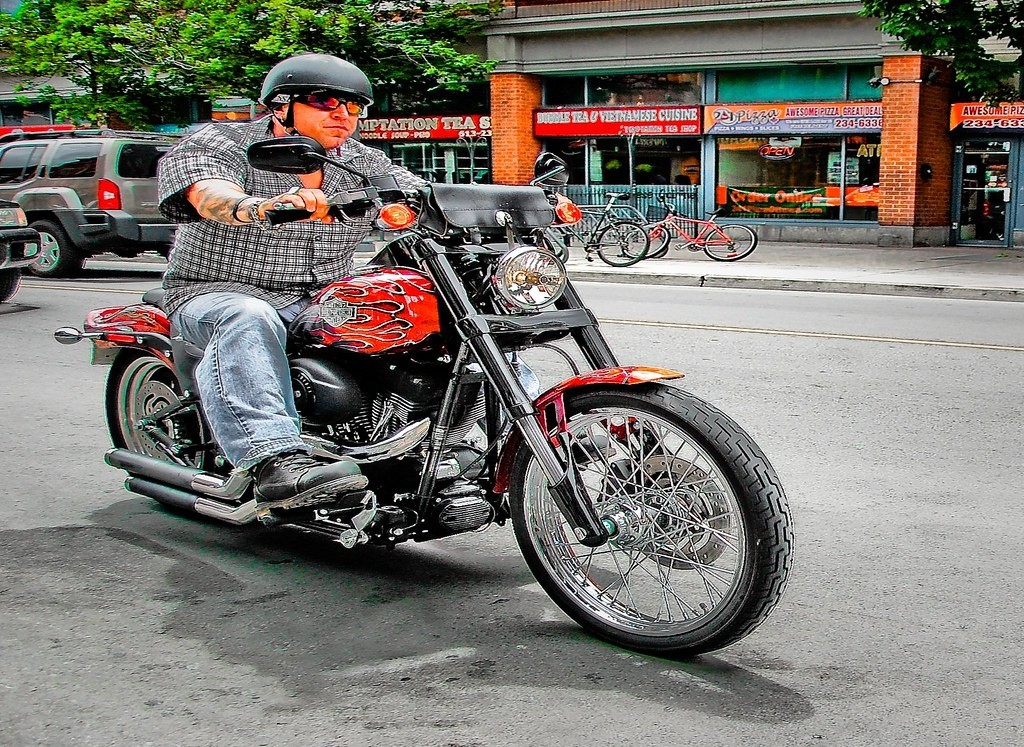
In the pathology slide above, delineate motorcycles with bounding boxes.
[52,135,796,658]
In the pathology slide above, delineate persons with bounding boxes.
[157,55,571,510]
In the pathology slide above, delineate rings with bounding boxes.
[290,187,301,194]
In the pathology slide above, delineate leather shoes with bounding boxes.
[248,448,369,518]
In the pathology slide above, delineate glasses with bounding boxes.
[290,91,366,115]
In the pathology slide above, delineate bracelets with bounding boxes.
[252,200,287,232]
[232,196,253,223]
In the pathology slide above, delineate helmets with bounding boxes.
[258,53,375,111]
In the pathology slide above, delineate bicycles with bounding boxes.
[621,189,758,262]
[537,191,651,268]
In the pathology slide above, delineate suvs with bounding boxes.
[0,198,42,305]
[2,128,181,279]
